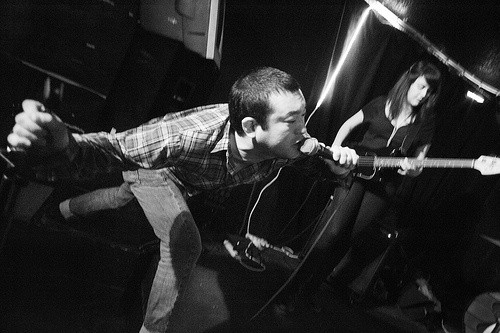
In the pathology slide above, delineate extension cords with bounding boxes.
[223,239,241,259]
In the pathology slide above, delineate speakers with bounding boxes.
[324,222,405,305]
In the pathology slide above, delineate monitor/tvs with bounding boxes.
[139,0,227,71]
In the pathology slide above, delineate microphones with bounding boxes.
[299,137,358,170]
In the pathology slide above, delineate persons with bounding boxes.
[6,65,361,333]
[268,59,443,316]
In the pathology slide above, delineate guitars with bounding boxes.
[356,146,500,184]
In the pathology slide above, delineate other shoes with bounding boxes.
[46,203,69,227]
[273,300,296,317]
[307,295,321,314]
